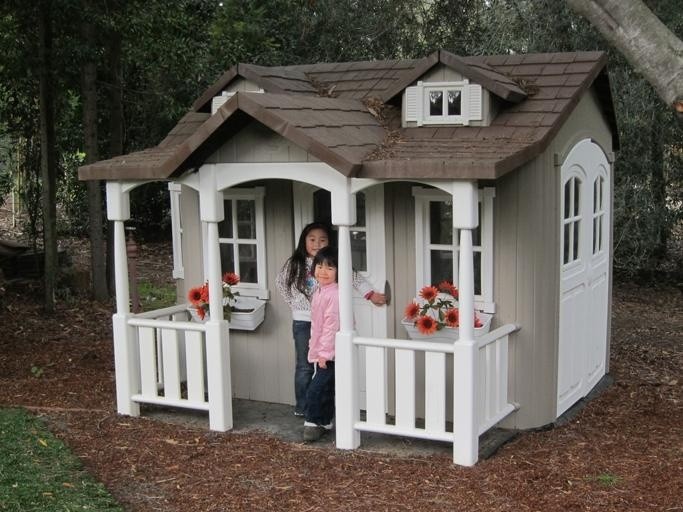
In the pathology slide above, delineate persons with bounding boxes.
[274,221,390,417]
[302,245,337,441]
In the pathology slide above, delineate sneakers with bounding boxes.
[302,424,330,441]
[295,415,305,425]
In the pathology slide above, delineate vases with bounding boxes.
[186,299,266,331]
[401,309,493,340]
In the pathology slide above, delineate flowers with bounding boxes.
[405,282,481,335]
[188,273,242,320]
